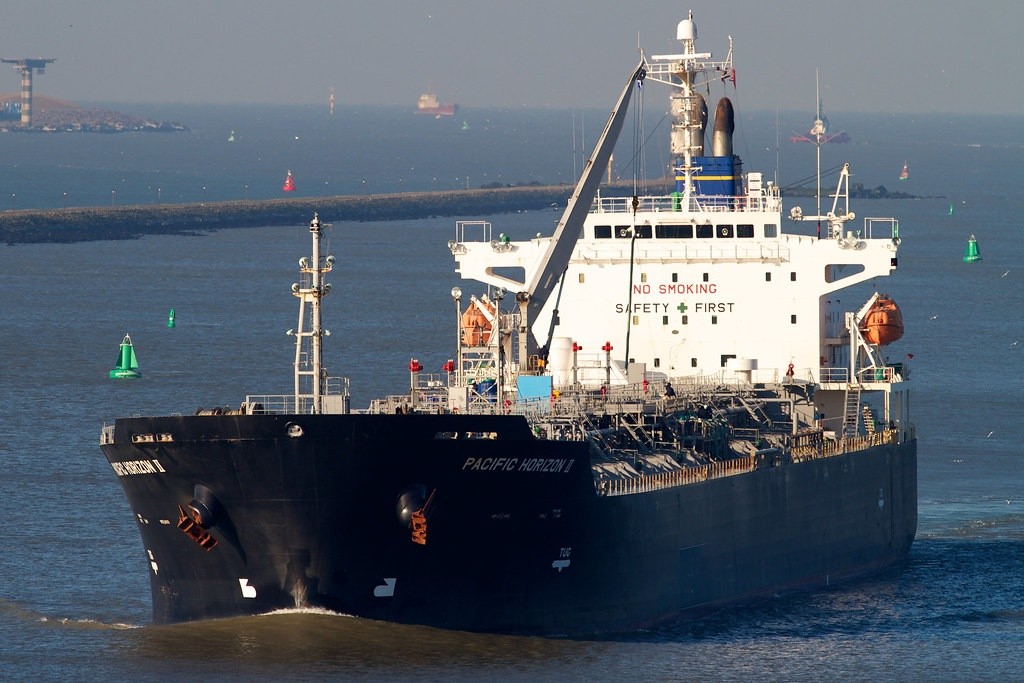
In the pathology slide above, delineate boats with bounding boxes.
[461,299,501,348]
[863,293,905,347]
[413,94,458,116]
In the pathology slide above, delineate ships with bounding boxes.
[91,8,922,643]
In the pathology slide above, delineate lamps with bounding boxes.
[722,228,728,236]
[620,227,632,237]
[499,233,510,244]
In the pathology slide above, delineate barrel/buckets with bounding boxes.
[466,378,496,402]
